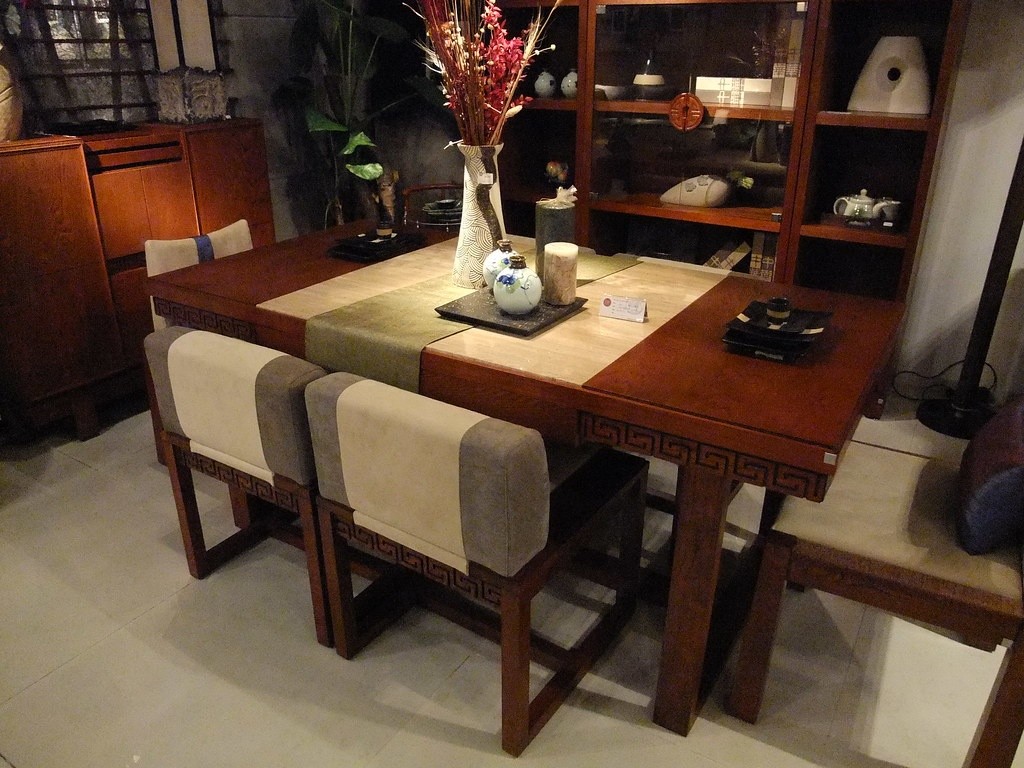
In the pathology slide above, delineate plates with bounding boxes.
[721,329,828,358]
[326,231,428,262]
[723,300,834,344]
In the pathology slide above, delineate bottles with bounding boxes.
[482,241,520,289]
[560,68,578,98]
[493,255,542,316]
[535,72,556,97]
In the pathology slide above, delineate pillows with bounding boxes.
[954,396,1024,555]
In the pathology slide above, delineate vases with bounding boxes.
[0,38,25,140]
[455,140,507,289]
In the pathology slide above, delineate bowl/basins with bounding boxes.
[436,199,456,209]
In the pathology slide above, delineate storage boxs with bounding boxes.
[702,231,777,283]
[696,63,801,109]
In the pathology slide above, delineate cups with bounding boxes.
[543,241,579,305]
[377,215,392,240]
[767,297,790,330]
[876,200,901,221]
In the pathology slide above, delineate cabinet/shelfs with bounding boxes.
[0,118,276,442]
[482,1,971,422]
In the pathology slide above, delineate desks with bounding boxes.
[147,218,908,738]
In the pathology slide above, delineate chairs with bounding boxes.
[143,325,387,647]
[303,372,652,758]
[145,219,255,330]
[728,393,1024,768]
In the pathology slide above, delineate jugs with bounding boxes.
[833,188,889,219]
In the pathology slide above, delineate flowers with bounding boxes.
[0,0,35,44]
[402,1,562,146]
